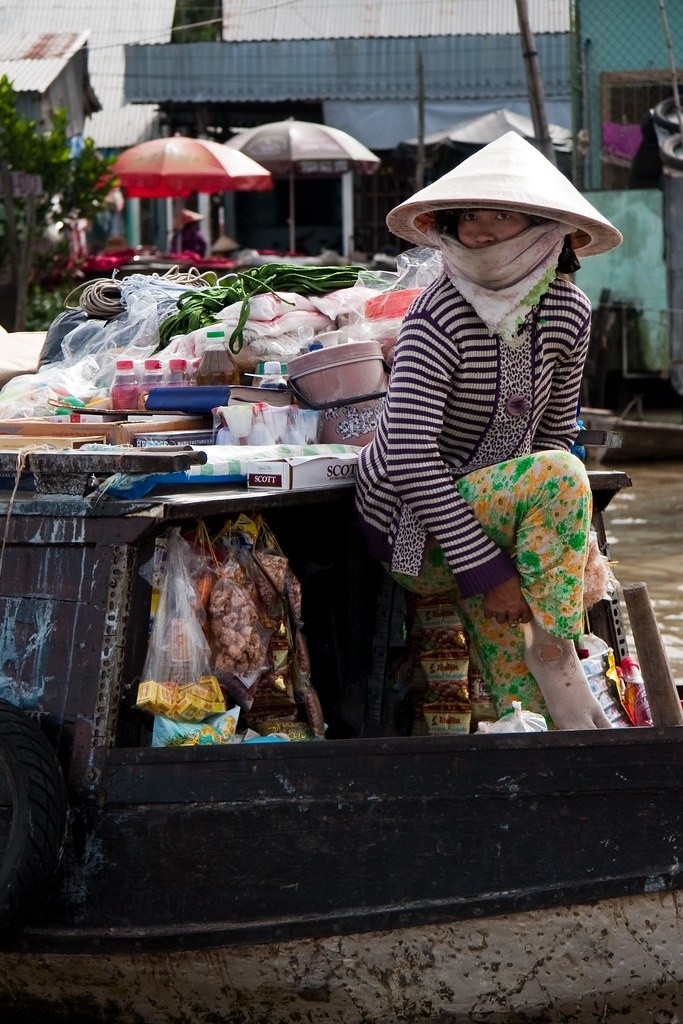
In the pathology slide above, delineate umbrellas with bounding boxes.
[398,110,572,153]
[97,132,272,253]
[224,117,381,254]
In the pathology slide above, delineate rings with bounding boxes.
[510,619,521,627]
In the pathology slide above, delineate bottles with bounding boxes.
[257,361,287,390]
[109,360,137,411]
[162,359,200,388]
[193,331,241,385]
[135,360,164,410]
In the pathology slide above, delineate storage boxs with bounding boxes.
[248,452,360,491]
[0,414,219,449]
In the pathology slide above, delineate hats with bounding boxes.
[174,208,205,231]
[386,131,623,258]
[210,236,239,253]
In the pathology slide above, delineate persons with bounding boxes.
[354,131,623,730]
[171,208,206,257]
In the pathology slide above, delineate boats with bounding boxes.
[0,401,682,1024]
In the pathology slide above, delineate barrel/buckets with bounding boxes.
[365,287,427,341]
[287,330,388,447]
